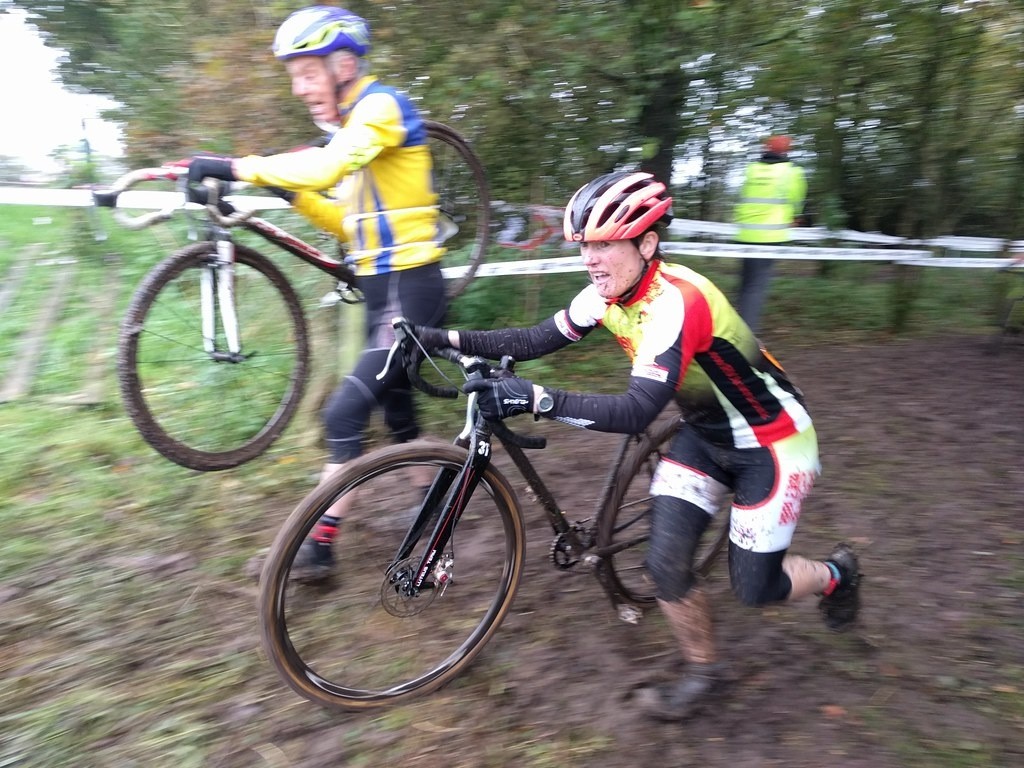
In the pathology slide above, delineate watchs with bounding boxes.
[531,382,555,415]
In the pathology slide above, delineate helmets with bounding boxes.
[562,170,673,242]
[271,5,374,60]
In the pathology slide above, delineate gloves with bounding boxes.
[400,325,452,369]
[258,149,300,202]
[186,155,233,204]
[461,369,535,421]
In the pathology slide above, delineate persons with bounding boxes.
[401,168,864,724]
[183,6,455,592]
[732,136,809,339]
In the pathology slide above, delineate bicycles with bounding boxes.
[88,119,493,470]
[255,317,737,713]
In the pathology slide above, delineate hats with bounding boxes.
[766,135,793,153]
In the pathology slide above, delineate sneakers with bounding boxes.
[817,540,863,635]
[244,544,337,583]
[406,514,453,540]
[637,661,739,724]
